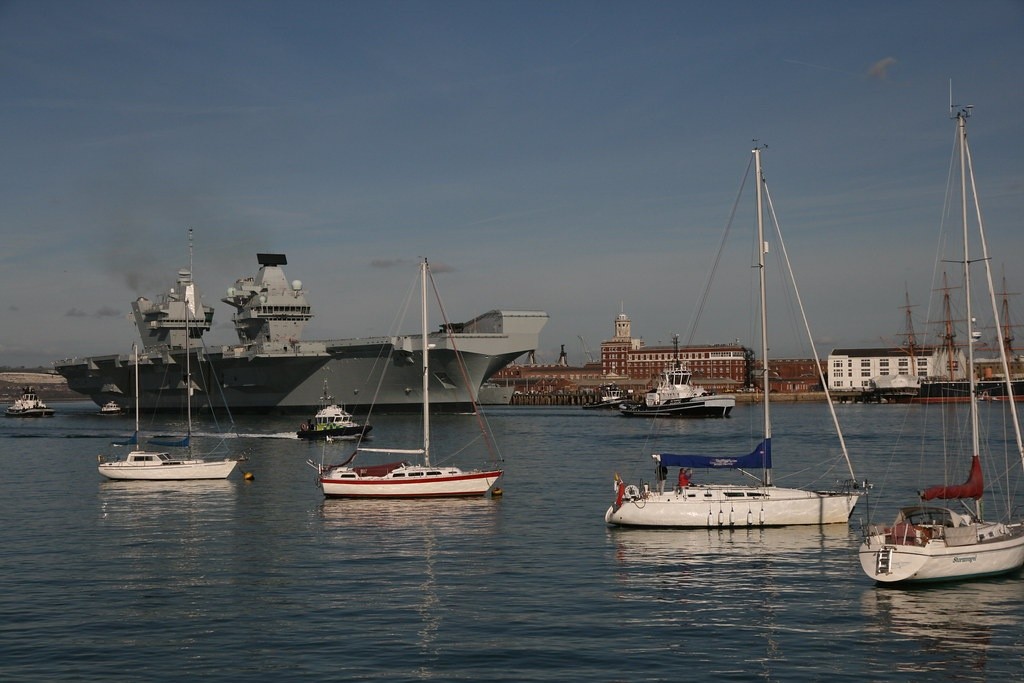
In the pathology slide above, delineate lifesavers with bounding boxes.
[493,488,501,493]
[625,484,639,498]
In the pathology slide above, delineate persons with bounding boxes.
[327,435,334,444]
[677,468,692,494]
[654,461,668,496]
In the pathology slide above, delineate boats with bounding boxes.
[294,378,375,445]
[3,384,56,416]
[492,486,503,496]
[99,399,122,414]
[52,226,554,417]
[618,329,736,417]
[582,384,641,410]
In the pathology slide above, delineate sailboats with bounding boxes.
[96,298,249,480]
[307,256,506,498]
[604,136,874,528]
[875,271,1024,404]
[858,102,1024,586]
